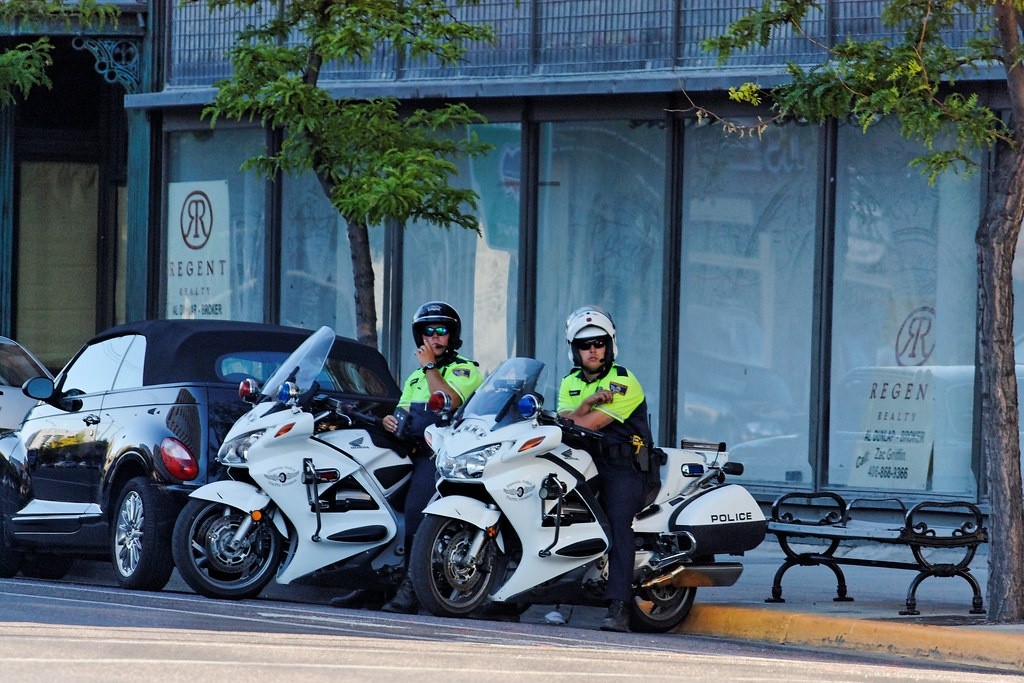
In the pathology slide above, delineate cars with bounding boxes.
[0,318,405,590]
[728,364,1024,483]
[0,337,55,430]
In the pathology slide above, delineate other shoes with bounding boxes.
[329,584,369,608]
[599,600,631,632]
[382,576,419,615]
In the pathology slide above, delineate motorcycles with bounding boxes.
[409,357,767,634]
[171,324,415,598]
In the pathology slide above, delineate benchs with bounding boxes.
[760,491,990,615]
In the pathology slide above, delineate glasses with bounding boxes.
[578,340,606,350]
[423,326,449,337]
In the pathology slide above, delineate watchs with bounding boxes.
[423,361,437,373]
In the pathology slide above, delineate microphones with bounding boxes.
[435,344,448,349]
[599,354,610,363]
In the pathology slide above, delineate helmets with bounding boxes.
[412,301,463,354]
[565,305,618,367]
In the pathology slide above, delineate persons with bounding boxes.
[330,302,482,613]
[557,306,660,632]
